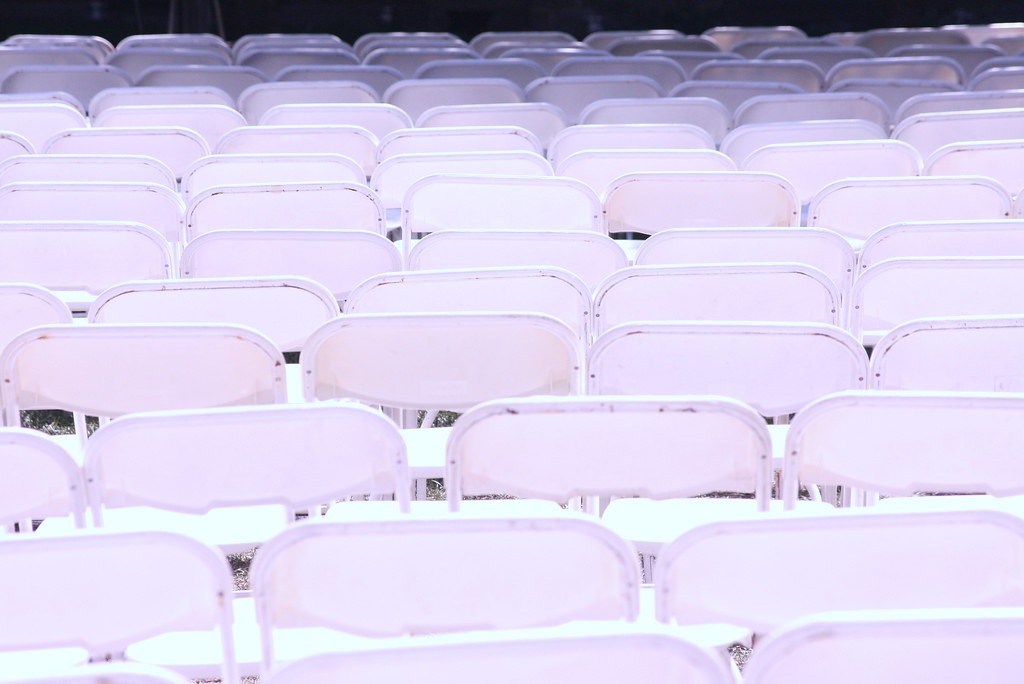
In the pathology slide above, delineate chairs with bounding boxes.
[0,25,1024,683]
